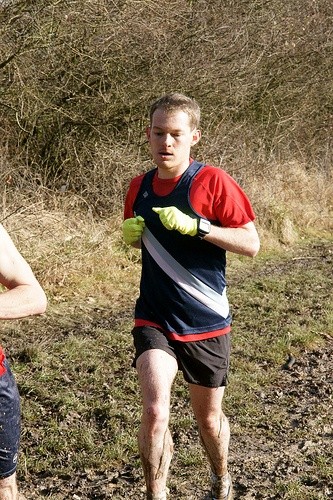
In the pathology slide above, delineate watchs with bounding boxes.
[193,215,212,241]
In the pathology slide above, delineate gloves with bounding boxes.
[152,201,198,238]
[122,215,146,247]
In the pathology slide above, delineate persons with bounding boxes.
[0,224,48,500]
[120,93,260,500]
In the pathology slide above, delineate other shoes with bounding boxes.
[208,476,234,500]
